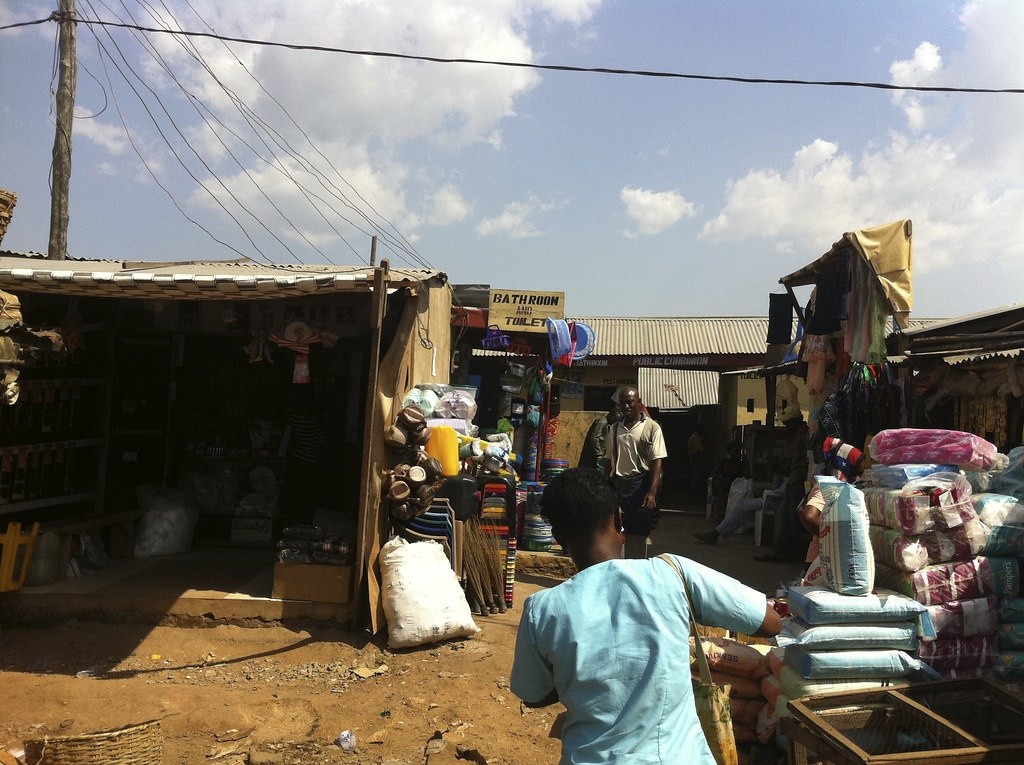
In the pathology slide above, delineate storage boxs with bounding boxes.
[230,516,278,542]
[270,558,355,605]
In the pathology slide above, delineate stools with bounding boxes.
[472,473,518,606]
[0,520,41,592]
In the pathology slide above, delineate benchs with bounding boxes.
[31,506,145,580]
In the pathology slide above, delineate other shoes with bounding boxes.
[693,531,719,544]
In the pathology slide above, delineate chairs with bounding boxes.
[753,491,783,547]
[388,498,455,570]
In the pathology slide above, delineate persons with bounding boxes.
[766,404,812,560]
[802,392,850,537]
[604,386,668,559]
[278,401,327,518]
[687,423,704,472]
[577,408,623,469]
[692,477,789,546]
[509,468,783,764]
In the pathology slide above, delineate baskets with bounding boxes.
[23,720,162,764]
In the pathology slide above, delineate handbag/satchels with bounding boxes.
[691,679,739,765]
[622,508,650,535]
